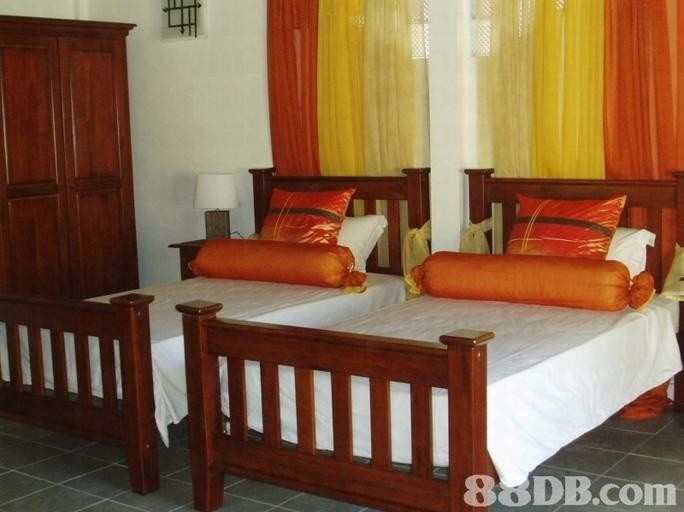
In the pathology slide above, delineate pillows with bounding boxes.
[187,239,366,288]
[606,227,657,276]
[260,187,357,245]
[505,193,629,261]
[341,214,388,270]
[409,252,655,313]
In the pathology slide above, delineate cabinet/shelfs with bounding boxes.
[0,14,138,306]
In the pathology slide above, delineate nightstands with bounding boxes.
[168,239,206,280]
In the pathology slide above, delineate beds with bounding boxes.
[176,166,684,512]
[0,165,431,494]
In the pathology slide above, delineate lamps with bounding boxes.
[193,174,241,238]
